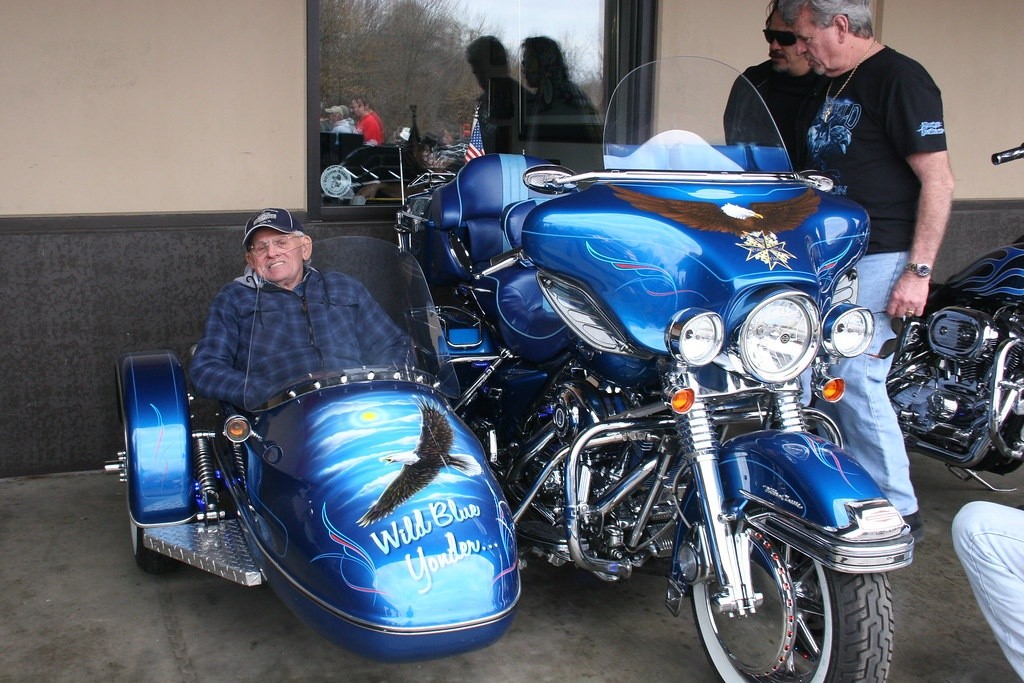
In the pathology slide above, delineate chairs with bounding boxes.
[430,156,572,359]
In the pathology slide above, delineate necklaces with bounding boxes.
[824,39,878,122]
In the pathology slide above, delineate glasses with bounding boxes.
[250,234,304,256]
[762,29,797,47]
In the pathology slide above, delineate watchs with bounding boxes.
[905,262,934,277]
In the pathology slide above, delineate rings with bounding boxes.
[907,310,914,314]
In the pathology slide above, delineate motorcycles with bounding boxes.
[94,53,918,683]
[882,144,1024,496]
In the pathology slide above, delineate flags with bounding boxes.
[464,121,484,164]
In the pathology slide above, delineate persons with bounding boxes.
[466,35,604,176]
[326,97,380,147]
[952,501,1024,681]
[722,0,825,171]
[790,0,955,542]
[188,206,418,411]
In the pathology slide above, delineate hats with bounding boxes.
[243,208,304,252]
[324,106,343,116]
